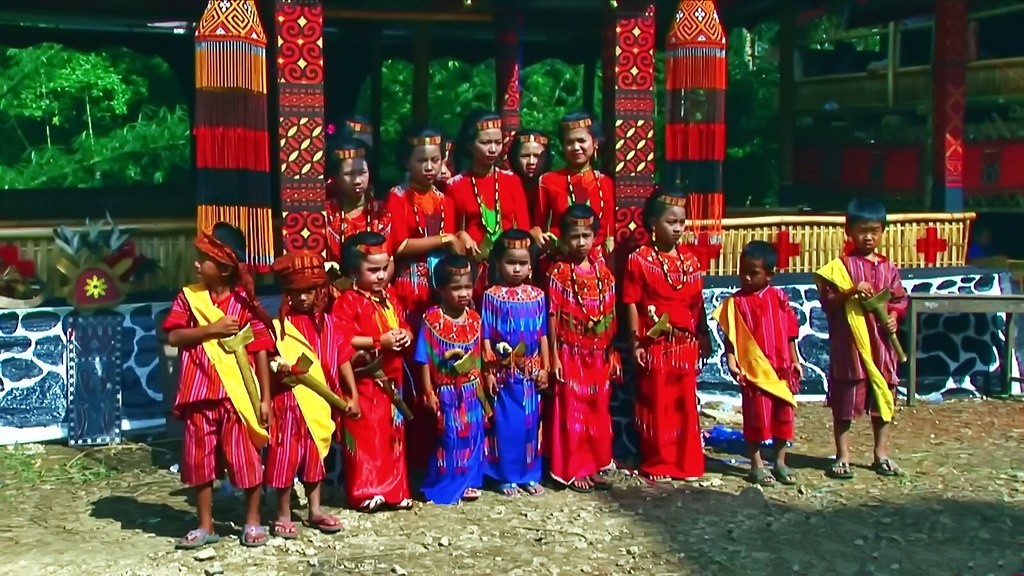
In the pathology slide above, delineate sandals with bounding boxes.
[391,498,413,510]
[361,495,385,513]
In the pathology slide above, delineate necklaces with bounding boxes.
[414,185,444,236]
[341,202,371,243]
[567,169,603,219]
[471,166,499,235]
[654,242,687,290]
[571,261,603,324]
[353,278,386,310]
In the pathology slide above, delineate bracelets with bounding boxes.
[542,364,550,373]
[422,391,435,407]
[484,367,496,375]
[372,336,382,358]
[441,234,451,246]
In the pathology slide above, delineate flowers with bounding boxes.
[0,243,36,281]
[52,210,146,310]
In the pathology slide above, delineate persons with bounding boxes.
[623,189,712,484]
[331,231,415,513]
[545,203,622,493]
[482,229,549,497]
[719,242,804,486]
[161,222,279,548]
[323,111,614,470]
[813,199,908,478]
[267,251,360,538]
[415,256,493,503]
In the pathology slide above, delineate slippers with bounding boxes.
[272,521,298,539]
[773,465,797,485]
[571,476,596,492]
[747,467,776,486]
[303,514,342,531]
[463,487,482,498]
[871,459,897,475]
[175,528,220,547]
[685,477,698,481]
[496,483,523,497]
[590,474,613,489]
[826,461,855,479]
[523,482,545,495]
[241,523,267,546]
[649,474,671,482]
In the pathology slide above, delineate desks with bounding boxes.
[0,289,179,445]
[906,293,1024,408]
